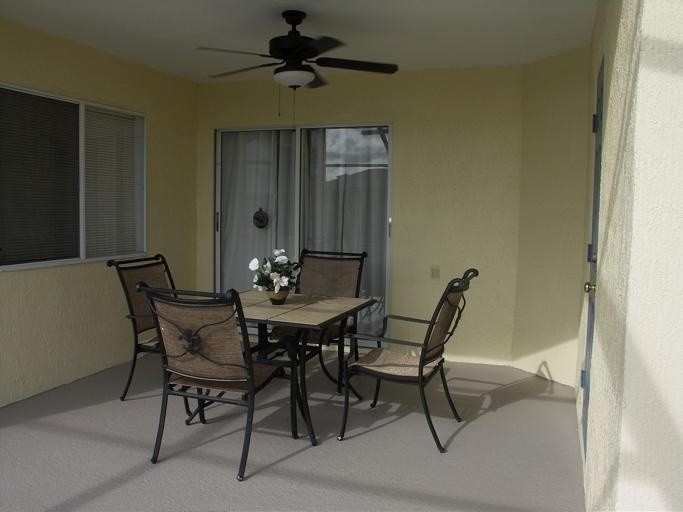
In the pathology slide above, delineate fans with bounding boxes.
[195,10,398,90]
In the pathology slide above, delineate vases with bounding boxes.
[266,289,289,306]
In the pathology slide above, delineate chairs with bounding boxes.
[338,269,479,453]
[260,249,367,393]
[106,255,194,416]
[134,281,300,481]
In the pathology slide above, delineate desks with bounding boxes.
[228,289,377,447]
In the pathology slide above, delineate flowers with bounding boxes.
[246,248,302,294]
